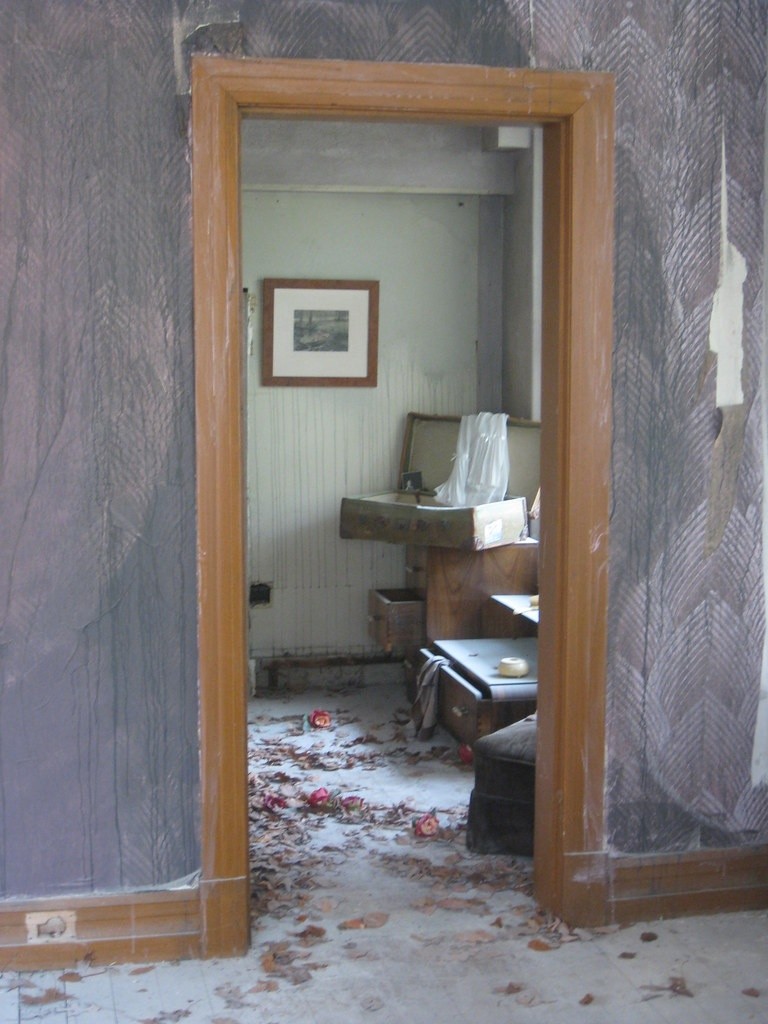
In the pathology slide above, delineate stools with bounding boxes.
[467,709,537,855]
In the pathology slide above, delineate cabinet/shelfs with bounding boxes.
[369,538,540,744]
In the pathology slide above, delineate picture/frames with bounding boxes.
[262,277,379,387]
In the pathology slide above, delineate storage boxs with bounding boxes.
[338,411,541,549]
